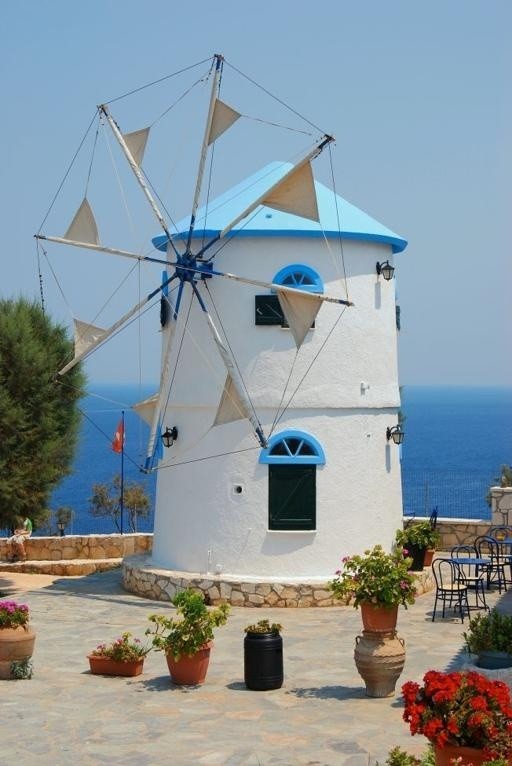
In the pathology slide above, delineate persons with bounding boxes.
[5,512,34,563]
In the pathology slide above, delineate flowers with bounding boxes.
[87,632,151,661]
[326,544,417,609]
[395,664,511,749]
[1,596,31,628]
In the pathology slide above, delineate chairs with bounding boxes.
[427,526,512,625]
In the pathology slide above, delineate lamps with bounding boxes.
[161,426,175,446]
[387,423,404,445]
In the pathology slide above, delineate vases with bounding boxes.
[87,655,143,676]
[358,600,397,635]
[428,743,494,766]
[0,624,37,681]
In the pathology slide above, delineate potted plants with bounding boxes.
[423,529,439,566]
[394,521,429,569]
[148,589,232,690]
[238,618,287,690]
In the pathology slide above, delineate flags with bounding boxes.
[112,418,125,454]
